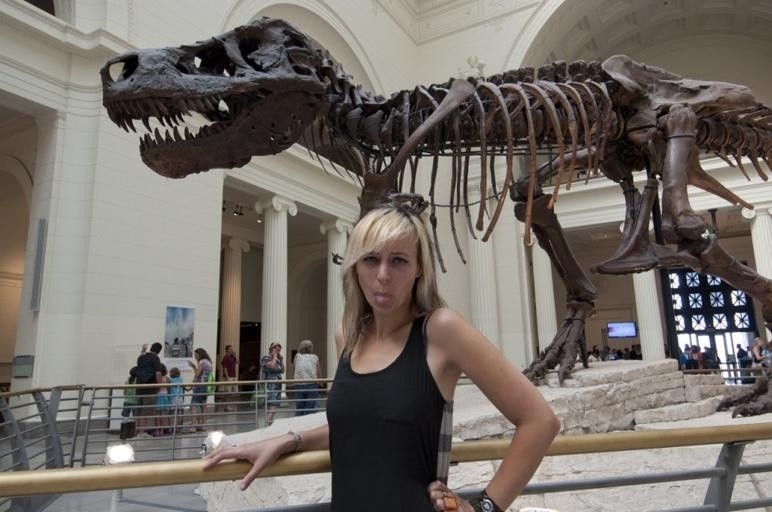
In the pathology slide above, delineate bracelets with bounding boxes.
[288,429,303,452]
[470,488,504,512]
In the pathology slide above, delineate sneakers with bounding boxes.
[137,425,208,440]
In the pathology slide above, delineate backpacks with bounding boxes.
[207,369,215,394]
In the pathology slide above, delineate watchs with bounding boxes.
[478,493,504,511]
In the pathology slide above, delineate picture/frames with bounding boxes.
[163,305,196,361]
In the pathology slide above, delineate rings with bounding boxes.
[232,443,237,448]
[441,490,447,498]
[443,496,458,510]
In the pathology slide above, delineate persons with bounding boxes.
[587,335,766,384]
[123,342,217,435]
[221,345,241,411]
[260,341,285,423]
[292,339,324,416]
[201,204,560,512]
